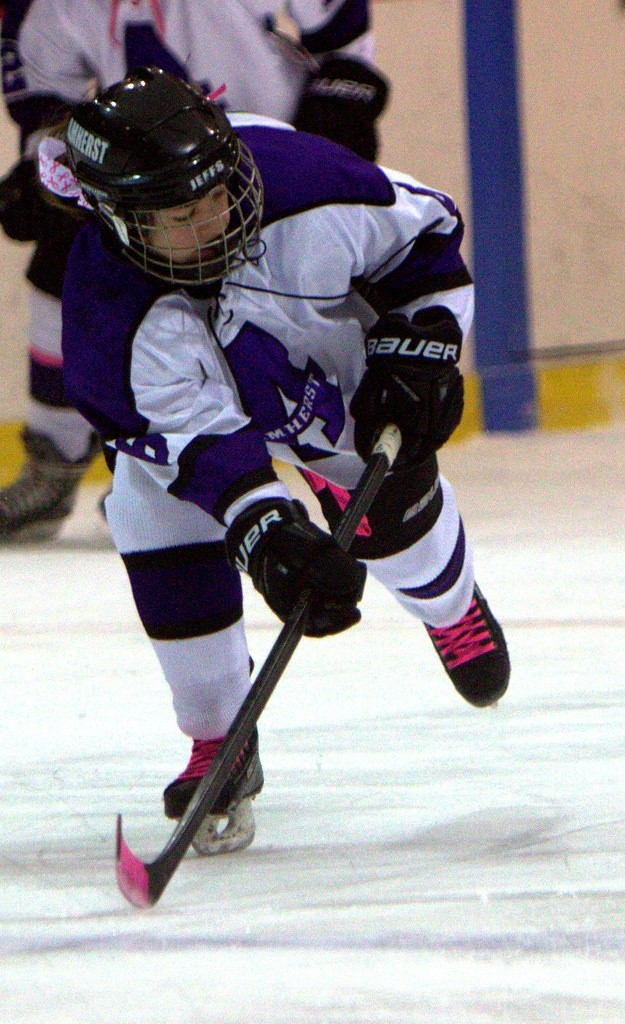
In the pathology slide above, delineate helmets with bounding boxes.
[65,67,240,209]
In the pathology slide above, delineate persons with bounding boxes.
[0,0,509,856]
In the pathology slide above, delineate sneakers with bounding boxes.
[423,582,510,712]
[163,724,264,856]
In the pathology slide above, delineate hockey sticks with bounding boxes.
[111,417,403,910]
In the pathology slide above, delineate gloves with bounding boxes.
[225,497,369,637]
[349,354,464,473]
